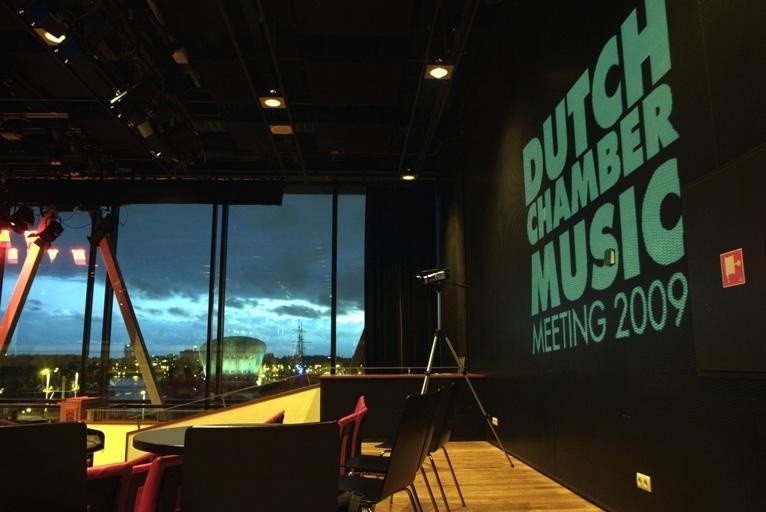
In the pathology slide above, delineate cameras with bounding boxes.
[414,268,450,287]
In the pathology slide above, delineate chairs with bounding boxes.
[338,393,436,512]
[134,421,338,511]
[392,388,465,511]
[2,422,131,512]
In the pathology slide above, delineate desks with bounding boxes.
[132,426,188,454]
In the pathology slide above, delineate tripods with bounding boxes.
[420,290,514,468]
